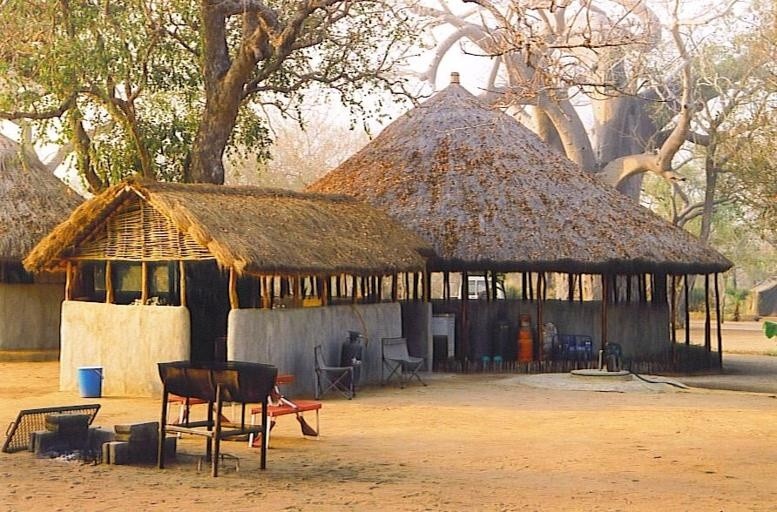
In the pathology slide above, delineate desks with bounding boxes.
[274,372,295,394]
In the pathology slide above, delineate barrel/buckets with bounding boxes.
[78,367,104,398]
[156,360,277,403]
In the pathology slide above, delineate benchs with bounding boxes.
[165,394,321,449]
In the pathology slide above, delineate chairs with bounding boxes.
[380,337,427,389]
[313,344,354,401]
